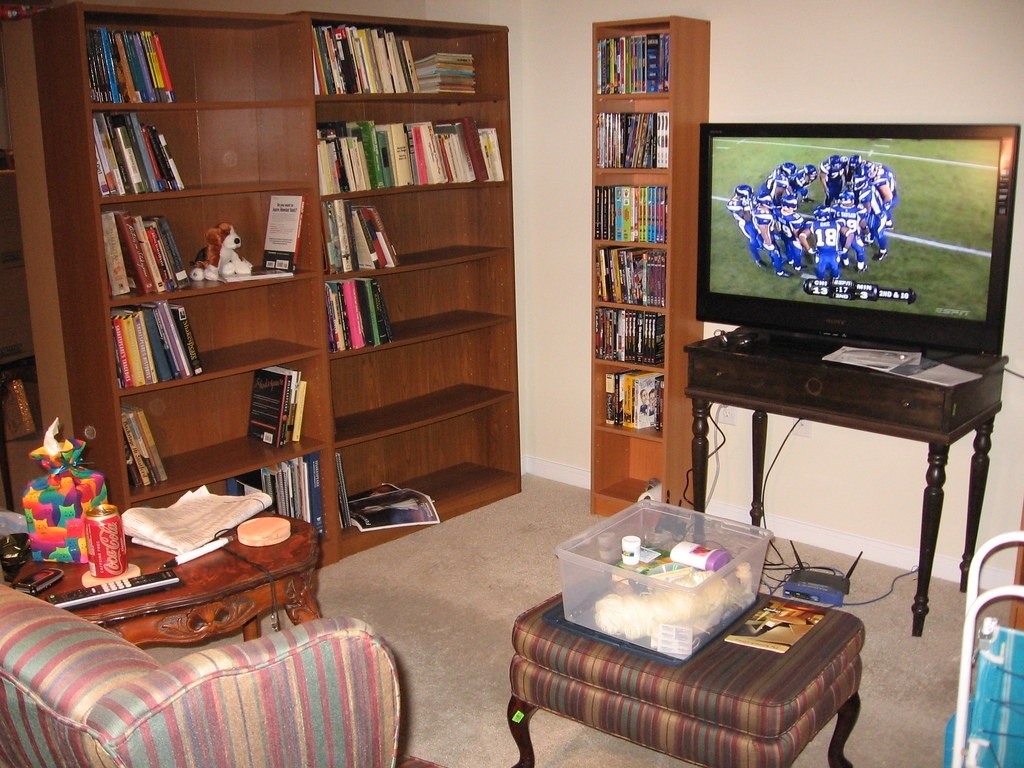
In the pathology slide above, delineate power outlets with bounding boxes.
[715,404,737,426]
[793,418,811,438]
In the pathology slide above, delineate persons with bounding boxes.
[727,154,899,281]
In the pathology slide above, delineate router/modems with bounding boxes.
[783,540,863,607]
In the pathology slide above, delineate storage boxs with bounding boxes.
[20,467,109,564]
[554,499,774,659]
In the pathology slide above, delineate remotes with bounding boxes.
[46,570,180,610]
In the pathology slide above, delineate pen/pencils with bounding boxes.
[156,534,236,570]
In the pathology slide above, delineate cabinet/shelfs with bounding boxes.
[682,326,1009,638]
[0,0,524,569]
[589,16,711,517]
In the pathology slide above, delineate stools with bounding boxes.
[506,588,867,767]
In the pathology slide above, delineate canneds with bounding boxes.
[83,504,128,579]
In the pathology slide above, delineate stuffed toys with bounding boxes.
[190,222,252,280]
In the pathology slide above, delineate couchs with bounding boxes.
[0,584,403,768]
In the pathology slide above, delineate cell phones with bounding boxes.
[12,569,64,596]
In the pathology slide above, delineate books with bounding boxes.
[605,369,665,431]
[325,278,407,353]
[597,34,670,94]
[336,452,441,533]
[595,309,665,365]
[87,29,176,103]
[596,248,666,307]
[317,116,508,196]
[262,195,307,271]
[93,113,186,194]
[261,457,311,523]
[120,402,168,487]
[595,186,667,243]
[111,301,202,388]
[321,199,399,274]
[248,365,308,447]
[596,112,669,168]
[101,208,191,294]
[313,24,476,95]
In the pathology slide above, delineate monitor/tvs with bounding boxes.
[696,123,1021,359]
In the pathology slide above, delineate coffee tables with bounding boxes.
[1,513,320,643]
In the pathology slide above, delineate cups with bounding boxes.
[622,536,641,564]
